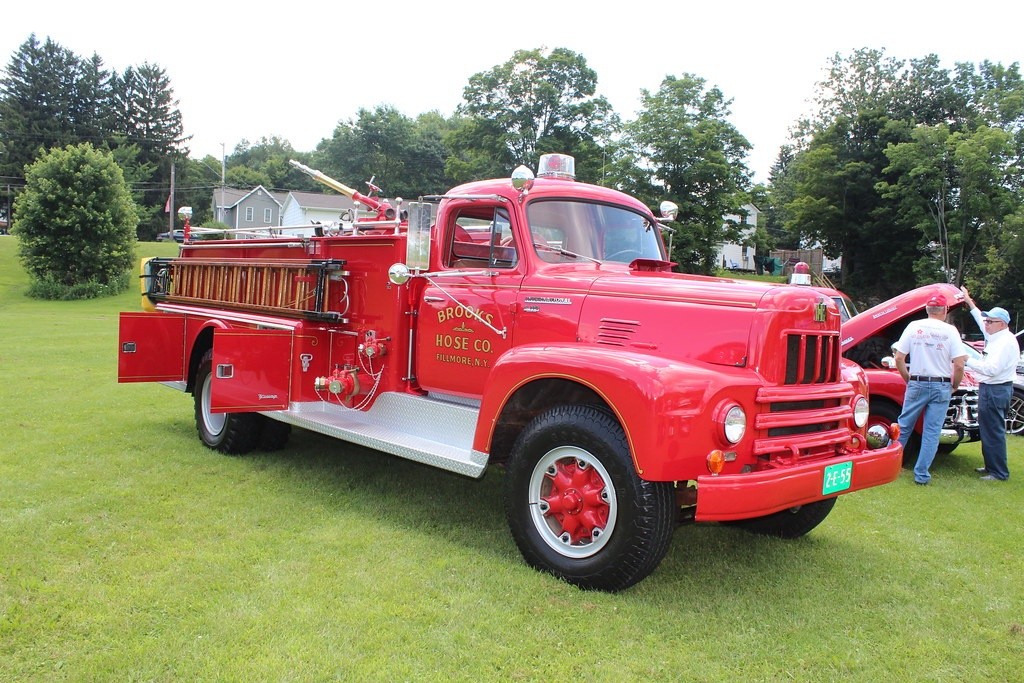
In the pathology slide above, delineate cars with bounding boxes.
[156,229,198,243]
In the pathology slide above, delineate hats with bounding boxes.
[981,307,1010,324]
[927,295,947,307]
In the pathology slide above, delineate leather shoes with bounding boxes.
[979,475,998,480]
[974,468,988,473]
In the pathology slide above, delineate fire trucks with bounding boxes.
[792,261,983,464]
[118,154,903,592]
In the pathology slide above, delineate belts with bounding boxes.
[1003,381,1013,386]
[910,375,952,382]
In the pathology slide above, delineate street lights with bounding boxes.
[197,143,225,223]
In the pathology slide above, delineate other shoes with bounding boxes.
[915,481,927,485]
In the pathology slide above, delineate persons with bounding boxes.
[961,285,1020,483]
[885,294,967,485]
[183,216,194,245]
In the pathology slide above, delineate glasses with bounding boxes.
[983,320,1002,324]
